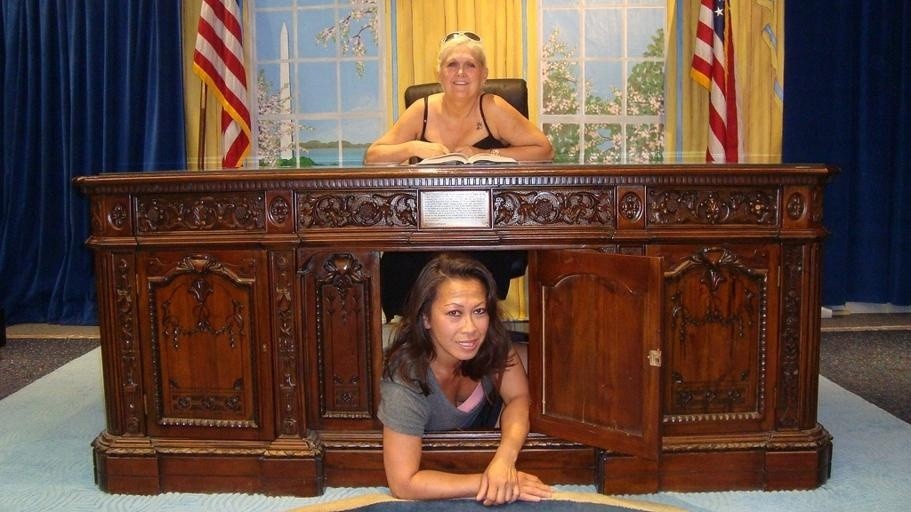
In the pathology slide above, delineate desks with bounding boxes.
[69,160,840,499]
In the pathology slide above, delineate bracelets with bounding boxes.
[489,148,501,155]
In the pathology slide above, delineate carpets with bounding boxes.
[2,343,911,512]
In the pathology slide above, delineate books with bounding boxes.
[416,153,518,165]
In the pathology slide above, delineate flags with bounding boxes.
[192,0,253,168]
[689,0,744,162]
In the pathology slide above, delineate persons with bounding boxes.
[376,253,556,506]
[364,32,555,323]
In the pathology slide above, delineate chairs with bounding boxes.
[403,78,529,166]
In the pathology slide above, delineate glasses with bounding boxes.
[444,32,480,42]
